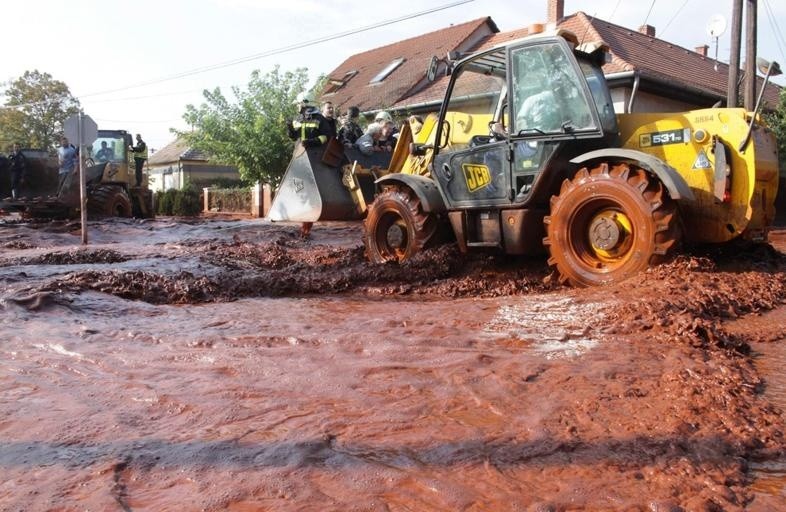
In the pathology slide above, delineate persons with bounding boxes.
[129,134,148,187]
[516,72,576,132]
[55,137,79,196]
[94,141,114,162]
[6,142,26,200]
[285,91,423,157]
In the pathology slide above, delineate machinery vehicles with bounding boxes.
[262,28,783,290]
[0,130,155,222]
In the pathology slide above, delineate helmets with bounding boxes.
[295,92,316,108]
[375,111,392,124]
